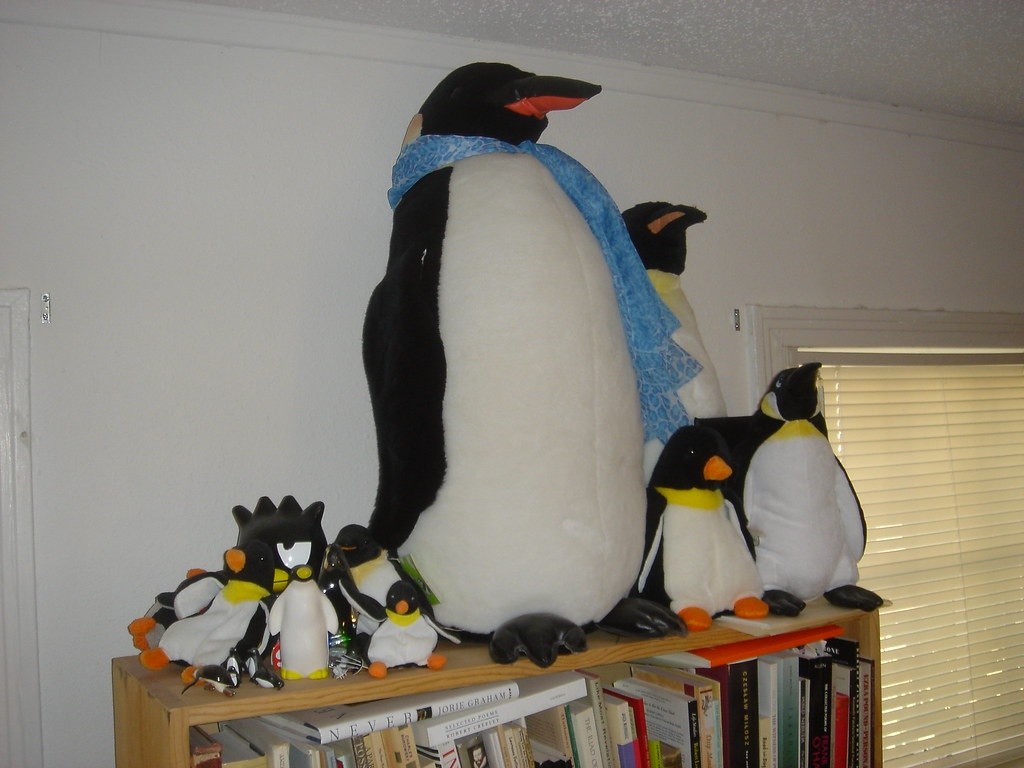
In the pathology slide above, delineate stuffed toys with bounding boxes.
[126,62,884,700]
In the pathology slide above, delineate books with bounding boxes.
[189,626,877,768]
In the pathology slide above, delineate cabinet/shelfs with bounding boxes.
[111,589,893,768]
